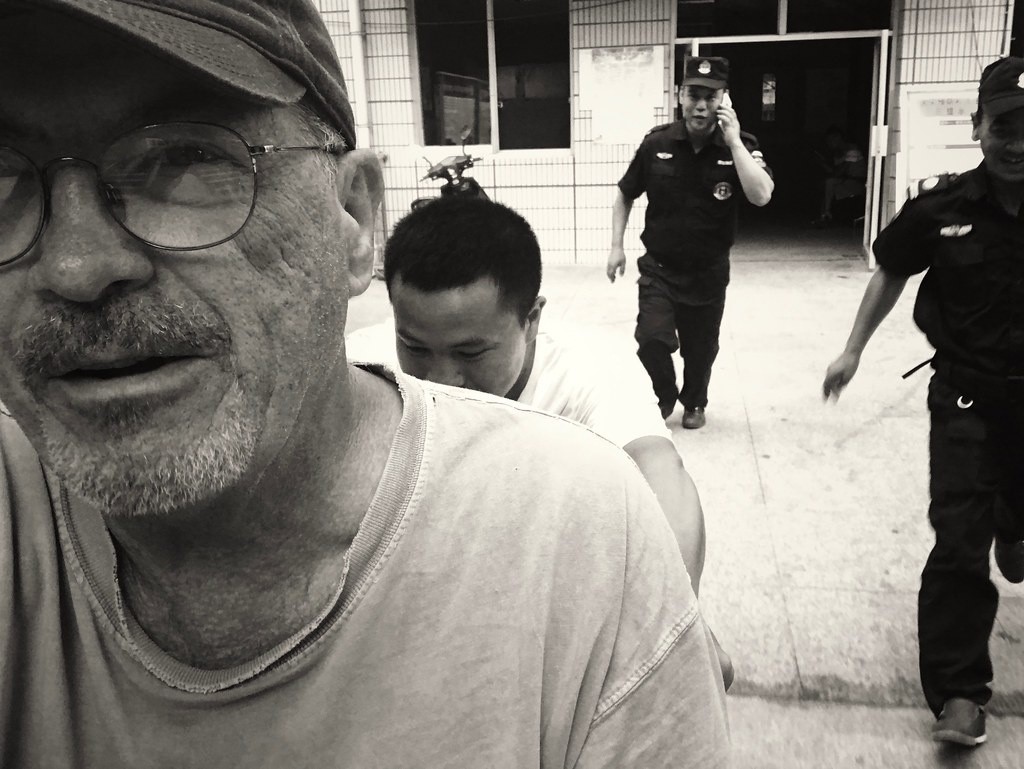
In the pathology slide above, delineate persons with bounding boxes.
[5,1,733,766]
[822,56,1022,746]
[384,198,734,687]
[609,58,775,431]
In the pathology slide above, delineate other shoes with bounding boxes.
[657,391,678,419]
[682,405,706,428]
[931,697,988,745]
[995,534,1024,584]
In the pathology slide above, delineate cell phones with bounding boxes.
[718,92,732,134]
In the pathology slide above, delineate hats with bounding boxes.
[979,55,1024,118]
[2,0,357,154]
[683,54,730,90]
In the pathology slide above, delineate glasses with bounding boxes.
[0,119,358,269]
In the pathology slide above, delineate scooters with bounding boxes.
[408,122,493,211]
